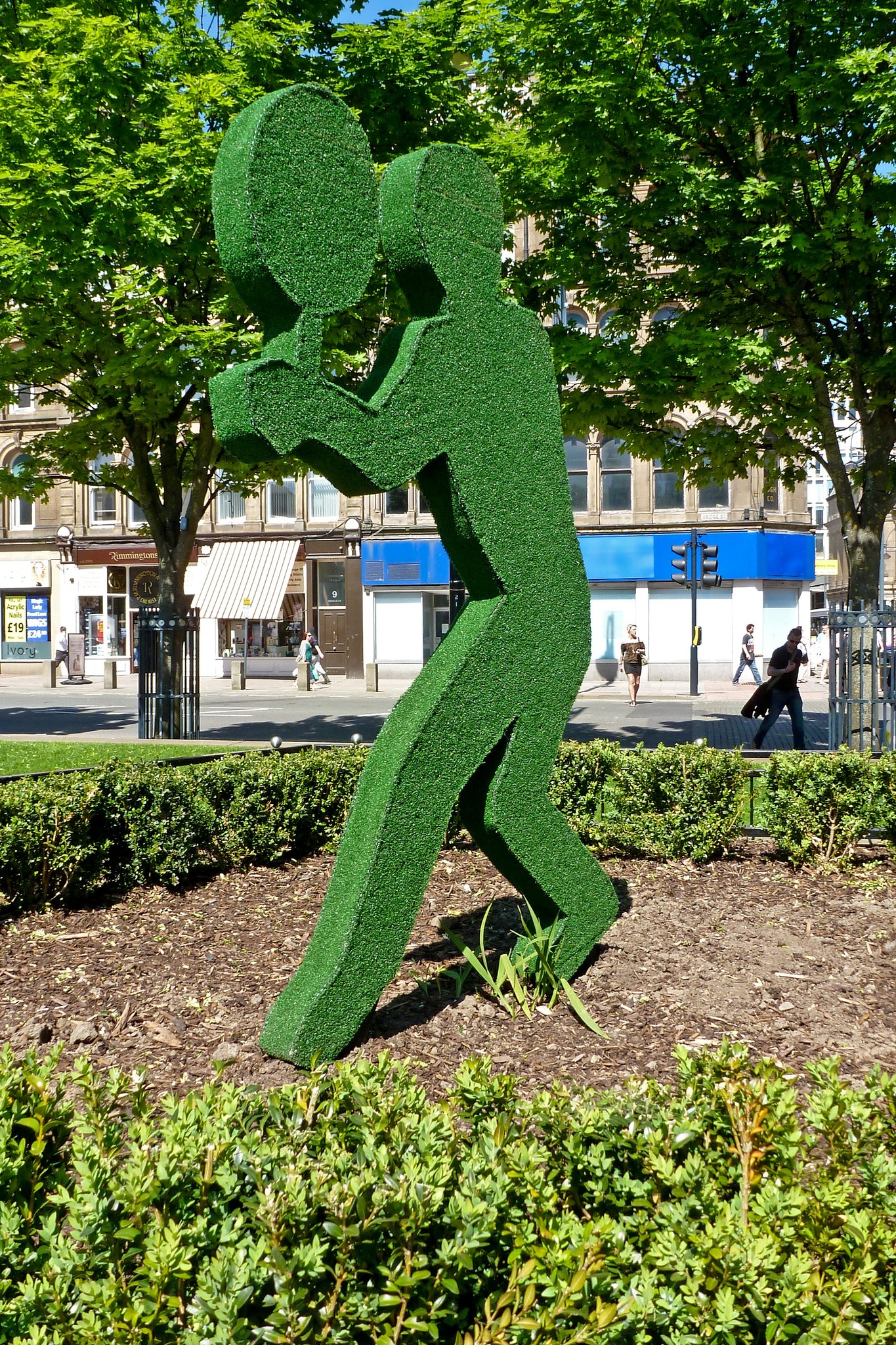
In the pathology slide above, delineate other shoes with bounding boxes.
[814,674,829,685]
[293,683,298,688]
[630,701,636,707]
[732,682,741,686]
[753,740,760,750]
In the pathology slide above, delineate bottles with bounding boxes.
[243,638,245,643]
[237,637,239,643]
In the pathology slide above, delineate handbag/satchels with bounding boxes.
[759,687,771,707]
[292,668,298,677]
[801,655,808,663]
[638,636,648,665]
[296,654,302,663]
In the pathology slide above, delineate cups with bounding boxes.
[262,651,281,657]
[225,653,227,657]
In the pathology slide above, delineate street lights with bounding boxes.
[844,456,884,612]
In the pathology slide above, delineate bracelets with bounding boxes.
[619,663,622,665]
[785,668,787,672]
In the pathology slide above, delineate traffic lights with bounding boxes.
[700,541,722,591]
[671,543,687,587]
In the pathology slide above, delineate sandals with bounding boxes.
[797,679,806,683]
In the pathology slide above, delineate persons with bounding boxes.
[292,632,330,686]
[796,626,809,683]
[810,625,840,685]
[753,629,804,751]
[54,626,68,670]
[619,624,646,707]
[32,561,47,583]
[732,624,764,686]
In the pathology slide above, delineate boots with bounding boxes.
[322,674,330,685]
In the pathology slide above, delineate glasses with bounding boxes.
[307,635,312,636]
[789,637,800,644]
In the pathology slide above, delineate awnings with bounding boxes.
[187,539,301,620]
